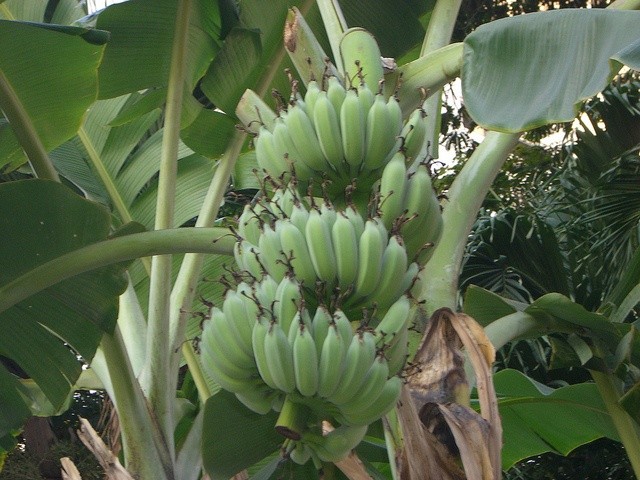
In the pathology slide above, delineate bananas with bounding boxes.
[198,68,444,465]
